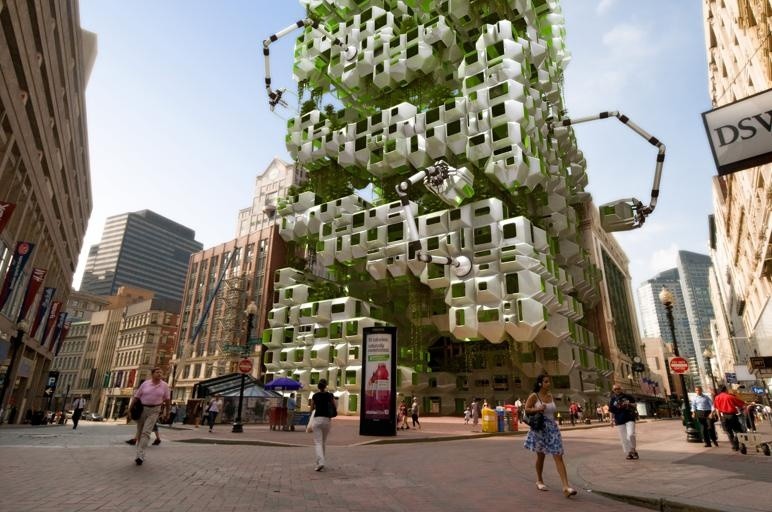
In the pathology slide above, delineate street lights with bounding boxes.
[232,301,257,432]
[167,358,181,401]
[703,348,718,396]
[658,284,701,443]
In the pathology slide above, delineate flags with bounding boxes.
[0,200,73,358]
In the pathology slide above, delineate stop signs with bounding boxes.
[238,359,254,374]
[669,356,690,375]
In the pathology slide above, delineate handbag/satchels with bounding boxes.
[130,397,143,421]
[523,409,544,431]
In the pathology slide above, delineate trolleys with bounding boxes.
[737,403,772,457]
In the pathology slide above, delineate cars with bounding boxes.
[46,408,103,422]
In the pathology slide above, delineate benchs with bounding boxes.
[292,414,311,432]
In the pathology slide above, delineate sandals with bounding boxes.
[563,487,576,498]
[626,451,639,460]
[536,482,548,491]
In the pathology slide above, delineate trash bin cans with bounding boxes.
[31,411,44,425]
[481,404,518,433]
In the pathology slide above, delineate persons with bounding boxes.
[126,366,172,466]
[310,378,339,470]
[166,402,176,429]
[692,384,771,451]
[123,378,161,447]
[398,398,421,429]
[523,373,579,501]
[70,392,87,430]
[287,392,300,431]
[464,399,488,424]
[597,403,610,422]
[608,385,639,459]
[201,394,222,432]
[570,400,583,424]
[515,397,526,423]
